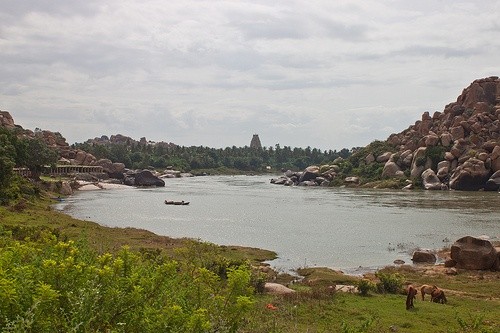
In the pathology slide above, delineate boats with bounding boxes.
[174,201,189,205]
[165,201,174,204]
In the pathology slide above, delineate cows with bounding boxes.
[407,286,418,298]
[421,285,436,302]
[433,289,447,304]
